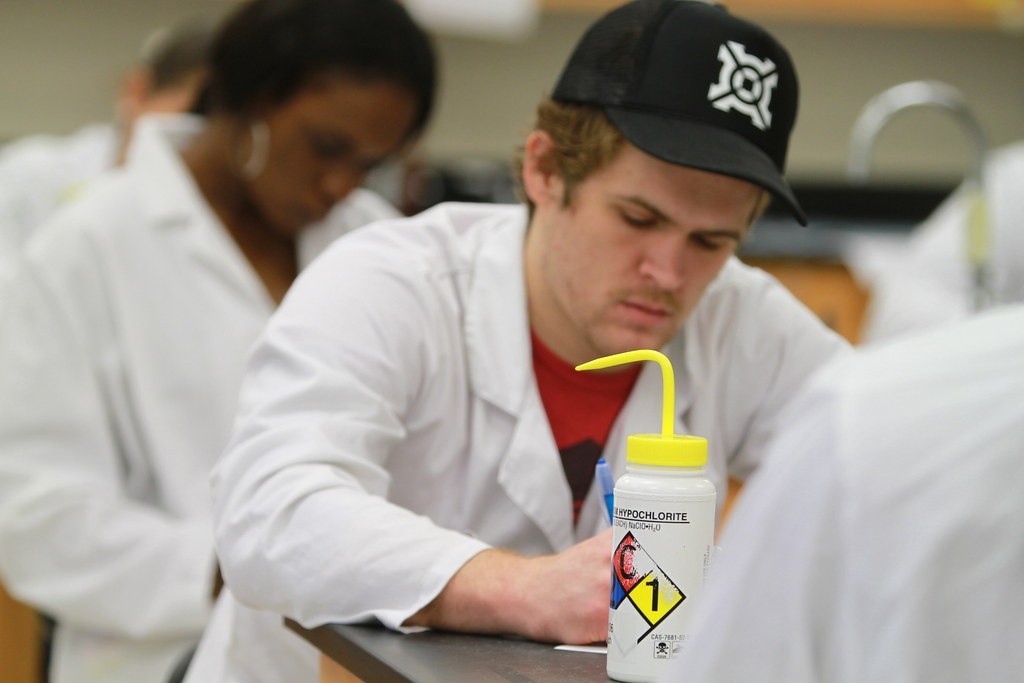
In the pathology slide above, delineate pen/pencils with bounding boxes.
[594,458,615,527]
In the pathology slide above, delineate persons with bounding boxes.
[175,2,853,683]
[0,17,220,248]
[867,135,1023,342]
[654,296,1024,683]
[2,2,442,683]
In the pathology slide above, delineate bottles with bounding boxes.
[575,349,718,682]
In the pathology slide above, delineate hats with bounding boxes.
[546,0,808,225]
